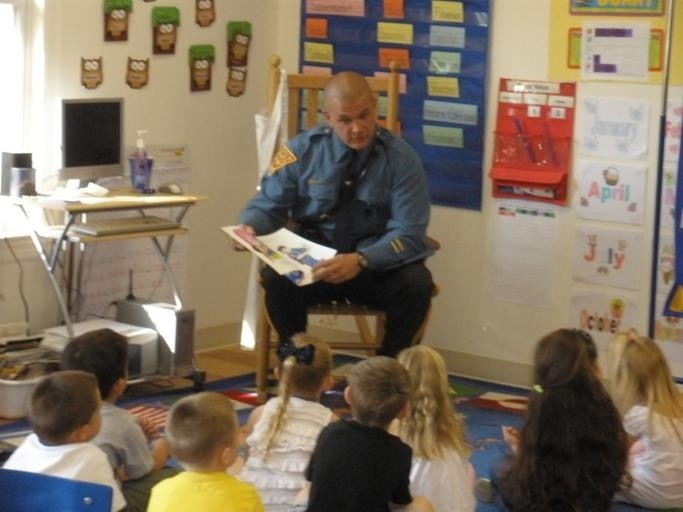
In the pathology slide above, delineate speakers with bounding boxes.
[1,152,32,195]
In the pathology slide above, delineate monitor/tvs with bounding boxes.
[52,97,125,199]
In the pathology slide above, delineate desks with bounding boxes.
[9,186,208,352]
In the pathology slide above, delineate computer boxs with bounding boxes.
[115,298,196,378]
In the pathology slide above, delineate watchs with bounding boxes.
[357,252,369,270]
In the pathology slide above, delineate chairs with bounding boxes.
[253,52,407,405]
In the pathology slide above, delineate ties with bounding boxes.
[340,151,358,204]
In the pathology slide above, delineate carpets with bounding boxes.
[0,352,682,511]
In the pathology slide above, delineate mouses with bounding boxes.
[158,183,184,194]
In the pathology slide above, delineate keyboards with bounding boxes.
[67,216,182,237]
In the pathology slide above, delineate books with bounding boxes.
[221,227,338,287]
[0,322,61,380]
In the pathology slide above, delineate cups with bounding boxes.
[127,157,155,189]
[10,167,35,197]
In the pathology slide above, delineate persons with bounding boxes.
[606,335,683,511]
[145,391,265,512]
[295,355,433,512]
[232,70,434,357]
[476,330,628,512]
[240,332,341,512]
[0,370,129,512]
[278,244,319,267]
[60,329,178,512]
[387,345,477,512]
